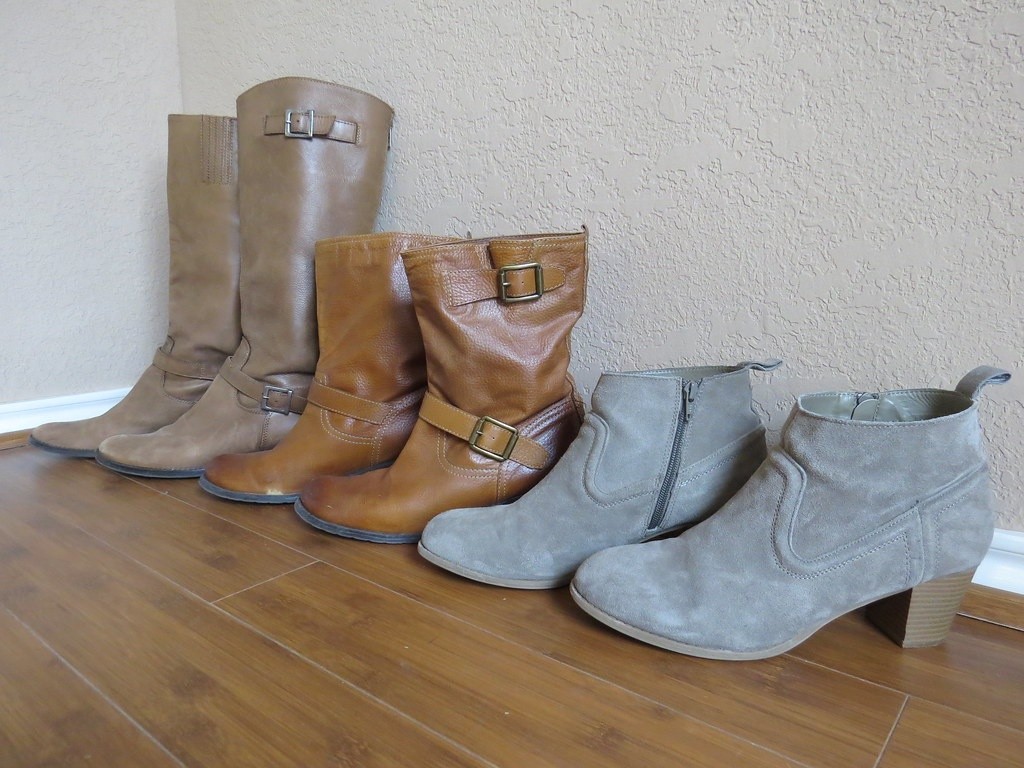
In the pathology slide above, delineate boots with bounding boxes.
[199,234,473,500]
[95,77,395,483]
[30,114,241,457]
[293,224,590,542]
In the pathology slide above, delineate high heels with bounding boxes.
[569,363,1010,660]
[417,359,781,591]
